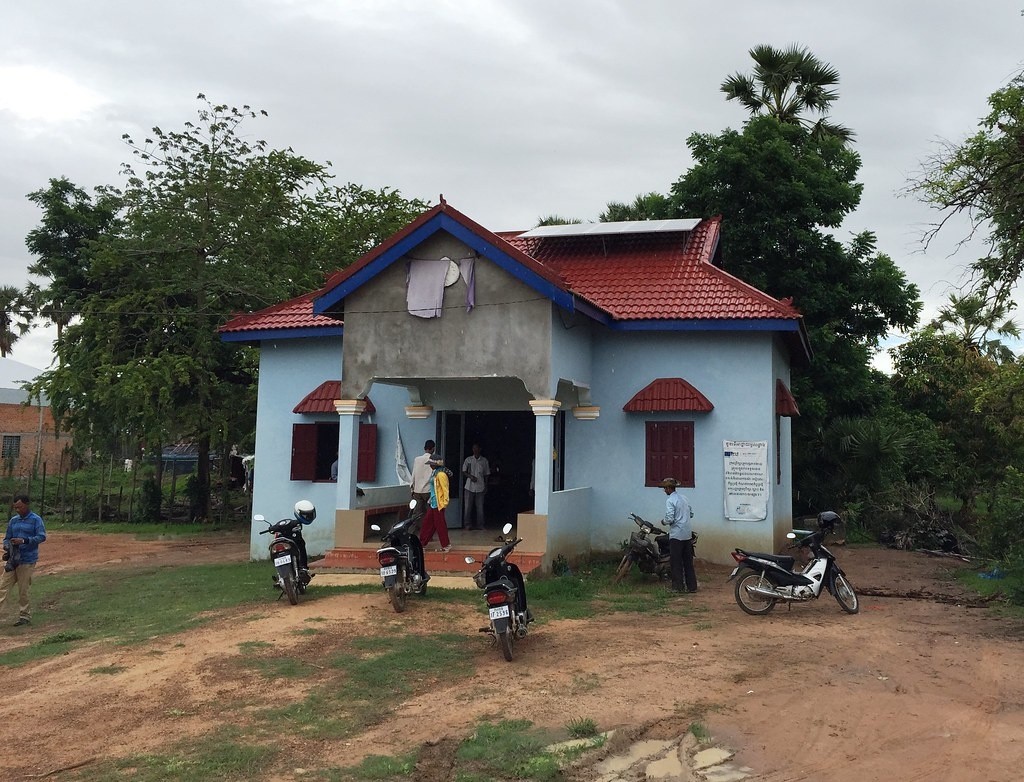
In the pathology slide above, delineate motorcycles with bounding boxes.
[615,512,699,585]
[726,511,859,615]
[464,523,534,662]
[254,500,316,605]
[370,499,430,612]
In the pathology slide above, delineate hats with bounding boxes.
[658,478,681,488]
[424,455,444,466]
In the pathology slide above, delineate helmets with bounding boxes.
[817,512,841,533]
[294,500,316,525]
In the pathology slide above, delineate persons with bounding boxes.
[409,440,436,533]
[462,442,491,531]
[0,495,46,626]
[331,452,338,480]
[529,448,535,510]
[658,477,698,593]
[419,454,452,552]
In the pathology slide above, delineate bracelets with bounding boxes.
[23,540,25,543]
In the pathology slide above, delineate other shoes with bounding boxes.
[442,545,452,552]
[13,619,29,627]
